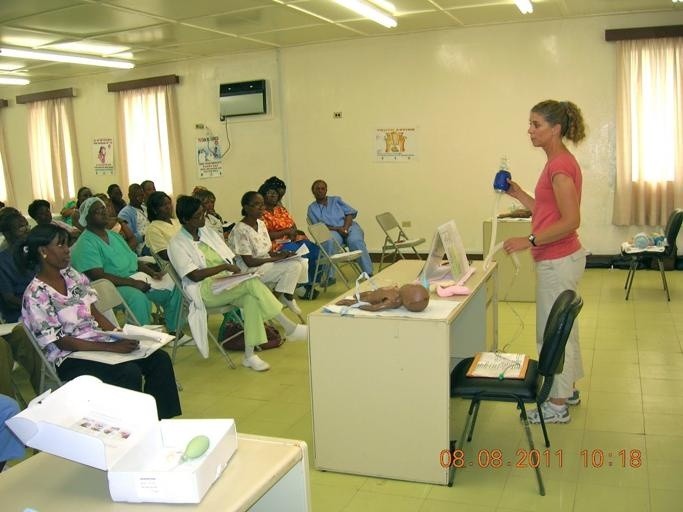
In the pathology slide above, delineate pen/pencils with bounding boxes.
[107,332,138,347]
[143,276,150,289]
[224,256,235,267]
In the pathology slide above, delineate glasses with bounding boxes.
[9,224,32,233]
[248,203,267,211]
[188,211,209,220]
[266,190,280,197]
[90,207,108,214]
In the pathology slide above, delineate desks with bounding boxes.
[481,218,539,302]
[0,433,311,511]
[307,261,500,487]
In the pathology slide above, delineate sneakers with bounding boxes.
[242,354,271,372]
[285,324,310,342]
[565,390,583,406]
[167,331,197,349]
[320,276,337,288]
[519,402,571,425]
[279,293,303,316]
[297,287,320,301]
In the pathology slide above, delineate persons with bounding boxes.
[141,180,156,203]
[503,101,586,423]
[168,196,308,372]
[28,200,79,247]
[147,192,183,261]
[307,180,374,287]
[1,207,40,324]
[117,184,152,256]
[335,284,428,312]
[73,187,92,231]
[228,191,309,314]
[59,200,76,227]
[93,193,137,249]
[13,224,181,420]
[267,176,286,200]
[70,197,184,340]
[108,184,127,215]
[0,393,27,474]
[259,184,319,300]
[192,186,236,242]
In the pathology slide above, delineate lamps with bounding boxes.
[0,46,135,69]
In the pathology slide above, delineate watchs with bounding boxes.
[528,234,536,247]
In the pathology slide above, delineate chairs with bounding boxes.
[375,212,426,273]
[307,222,367,301]
[618,208,683,302]
[305,217,367,273]
[450,287,583,496]
[165,261,277,370]
[277,305,307,327]
[20,318,63,393]
[88,278,185,396]
